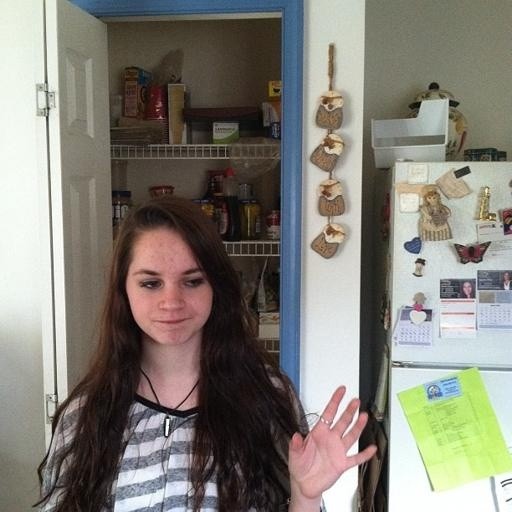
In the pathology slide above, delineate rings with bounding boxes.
[320,415,333,425]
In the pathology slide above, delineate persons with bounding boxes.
[418,191,451,241]
[460,281,475,298]
[32,192,377,512]
[500,271,512,290]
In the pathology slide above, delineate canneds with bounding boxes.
[111,190,132,242]
[238,183,280,241]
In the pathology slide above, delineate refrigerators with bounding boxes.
[372,162,511,512]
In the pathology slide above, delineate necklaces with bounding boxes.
[139,368,200,436]
